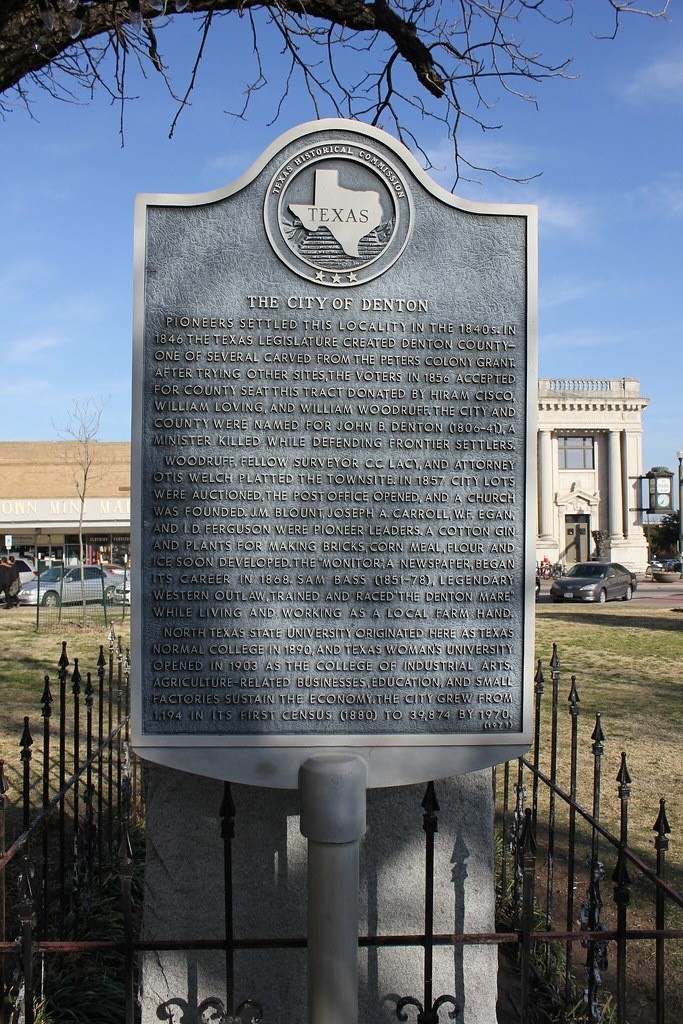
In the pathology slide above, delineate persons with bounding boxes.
[0,556,22,609]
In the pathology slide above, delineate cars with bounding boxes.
[661,560,678,567]
[104,565,130,581]
[0,559,39,600]
[112,583,130,606]
[652,561,663,568]
[549,561,637,603]
[15,564,125,608]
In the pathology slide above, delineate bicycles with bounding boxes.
[536,562,569,580]
